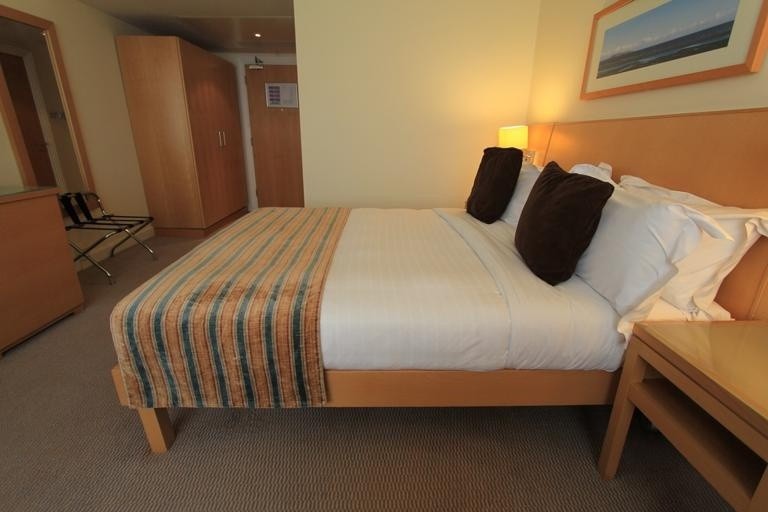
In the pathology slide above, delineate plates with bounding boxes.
[114,34,250,238]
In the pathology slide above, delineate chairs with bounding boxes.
[57,191,158,285]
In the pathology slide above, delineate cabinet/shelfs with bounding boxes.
[114,34,250,238]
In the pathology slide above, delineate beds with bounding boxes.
[107,205,736,453]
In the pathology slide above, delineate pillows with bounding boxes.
[575,188,734,339]
[465,146,522,223]
[618,176,768,322]
[504,161,620,228]
[515,162,615,286]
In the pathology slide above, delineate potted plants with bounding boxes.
[578,0,768,101]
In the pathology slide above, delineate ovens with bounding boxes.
[107,205,736,453]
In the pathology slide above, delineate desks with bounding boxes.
[0,184,85,358]
[594,319,768,512]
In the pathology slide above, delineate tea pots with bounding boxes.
[0,4,101,218]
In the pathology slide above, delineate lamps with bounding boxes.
[498,125,529,150]
[57,191,158,285]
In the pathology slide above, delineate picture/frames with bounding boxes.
[0,184,85,358]
[578,0,768,101]
[594,319,768,512]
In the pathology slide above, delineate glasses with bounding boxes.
[498,125,529,150]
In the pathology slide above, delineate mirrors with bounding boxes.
[0,4,101,218]
